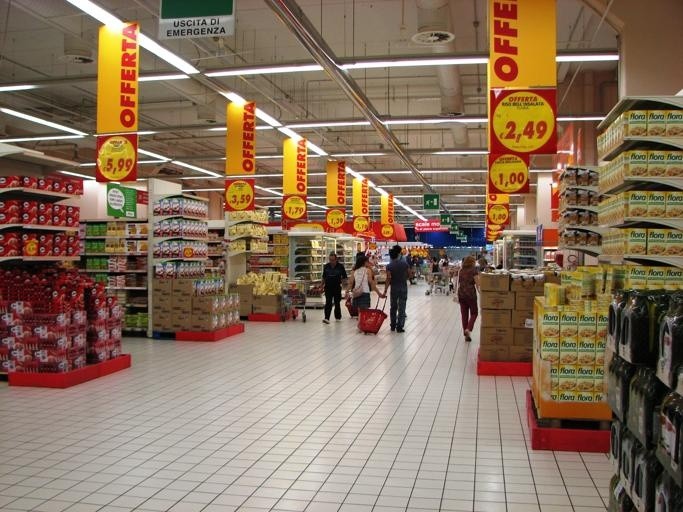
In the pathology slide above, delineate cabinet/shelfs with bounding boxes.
[596,96,682,269]
[493,231,559,268]
[557,165,602,256]
[247,230,363,307]
[0,144,81,263]
[206,211,270,271]
[80,178,210,338]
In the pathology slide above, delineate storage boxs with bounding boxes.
[479,269,561,362]
[228,283,286,316]
[153,278,240,332]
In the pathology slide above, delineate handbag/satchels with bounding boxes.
[353,285,363,298]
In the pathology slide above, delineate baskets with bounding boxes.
[358,309,387,333]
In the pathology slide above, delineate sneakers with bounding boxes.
[464,329,471,342]
[391,325,404,332]
[323,318,342,324]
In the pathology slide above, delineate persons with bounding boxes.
[348,245,407,332]
[407,248,492,285]
[318,252,348,324]
[457,256,480,342]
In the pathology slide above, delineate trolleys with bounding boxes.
[278,276,306,322]
[424,272,449,296]
[357,293,387,334]
[345,291,367,317]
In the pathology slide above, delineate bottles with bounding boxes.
[604,287,683,512]
